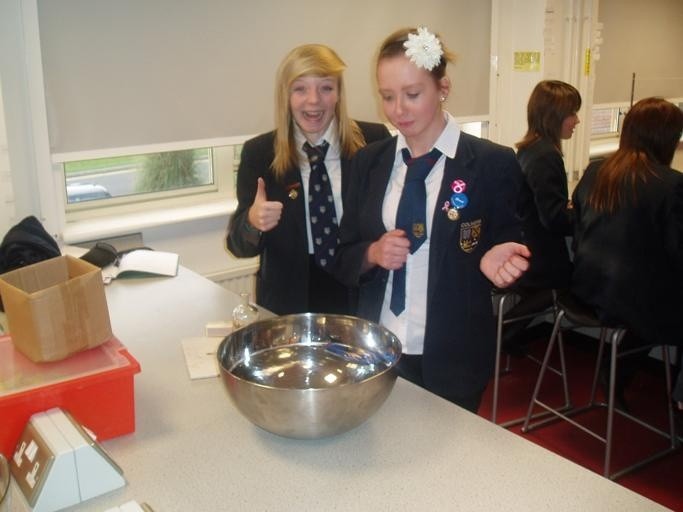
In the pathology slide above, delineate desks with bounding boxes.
[0,251,683,512]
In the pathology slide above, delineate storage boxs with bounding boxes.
[0,315,140,461]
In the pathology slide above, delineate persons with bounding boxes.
[329,25,552,415]
[494,78,582,356]
[569,97,682,438]
[227,44,393,318]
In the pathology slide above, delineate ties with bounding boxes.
[388,147,442,317]
[302,140,343,277]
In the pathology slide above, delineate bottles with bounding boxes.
[231,291,259,328]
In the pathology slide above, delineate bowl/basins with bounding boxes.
[215,313,399,441]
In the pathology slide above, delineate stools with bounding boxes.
[479,274,575,430]
[522,290,682,482]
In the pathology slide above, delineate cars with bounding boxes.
[65,182,110,203]
